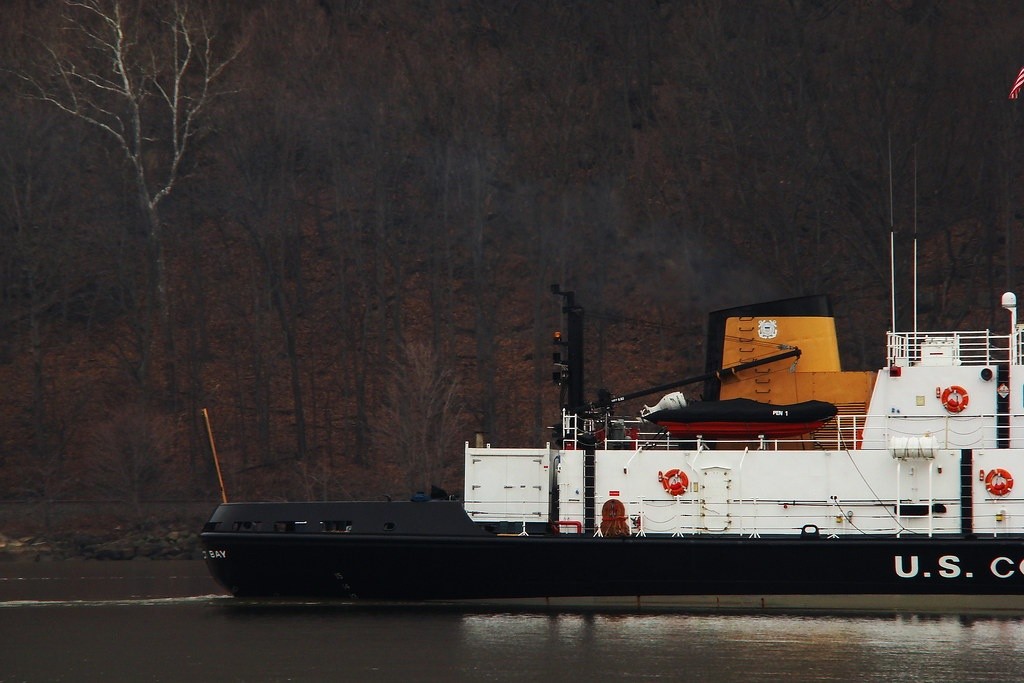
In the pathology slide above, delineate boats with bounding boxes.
[201,128,1024,616]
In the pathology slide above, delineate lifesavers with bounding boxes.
[985,469,1013,495]
[662,468,689,494]
[942,386,969,413]
